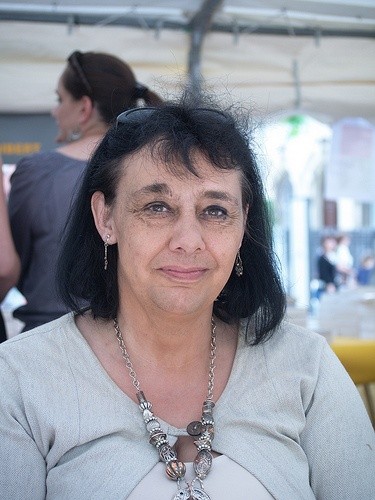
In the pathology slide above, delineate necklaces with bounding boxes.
[112,313,216,500]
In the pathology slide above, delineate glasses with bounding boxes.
[68,50,95,106]
[114,107,234,131]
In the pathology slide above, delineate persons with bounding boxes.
[316,233,375,300]
[0,51,162,342]
[0,100,375,500]
